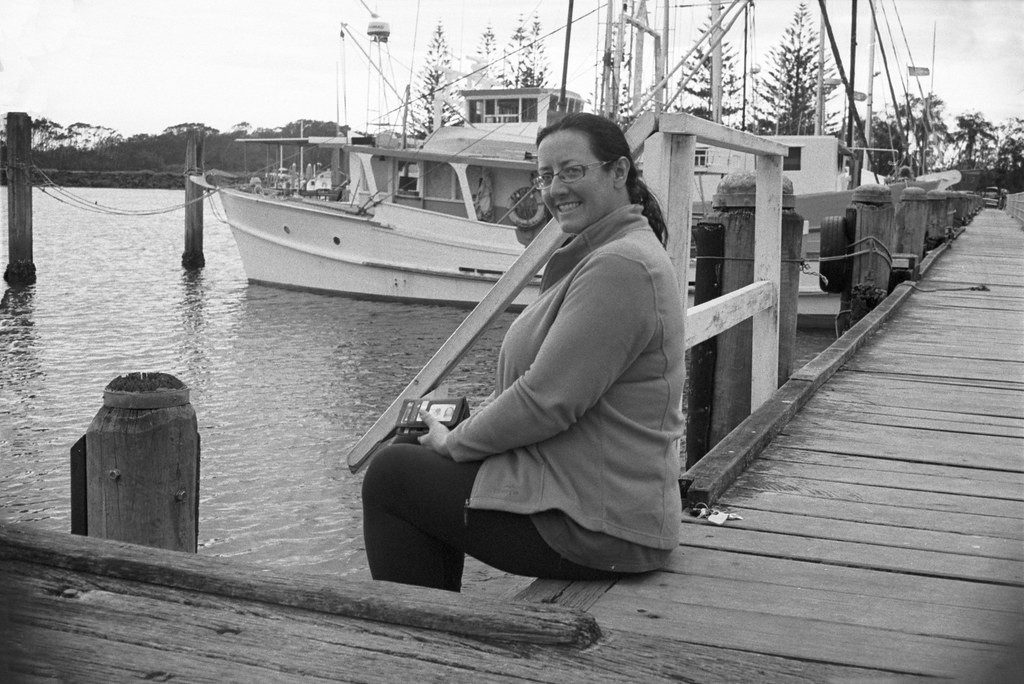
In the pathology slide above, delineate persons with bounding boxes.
[362,114,686,593]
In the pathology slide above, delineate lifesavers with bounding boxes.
[817,214,848,295]
[507,187,548,227]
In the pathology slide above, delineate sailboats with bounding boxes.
[192,1,961,327]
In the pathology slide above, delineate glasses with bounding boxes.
[532,160,613,190]
